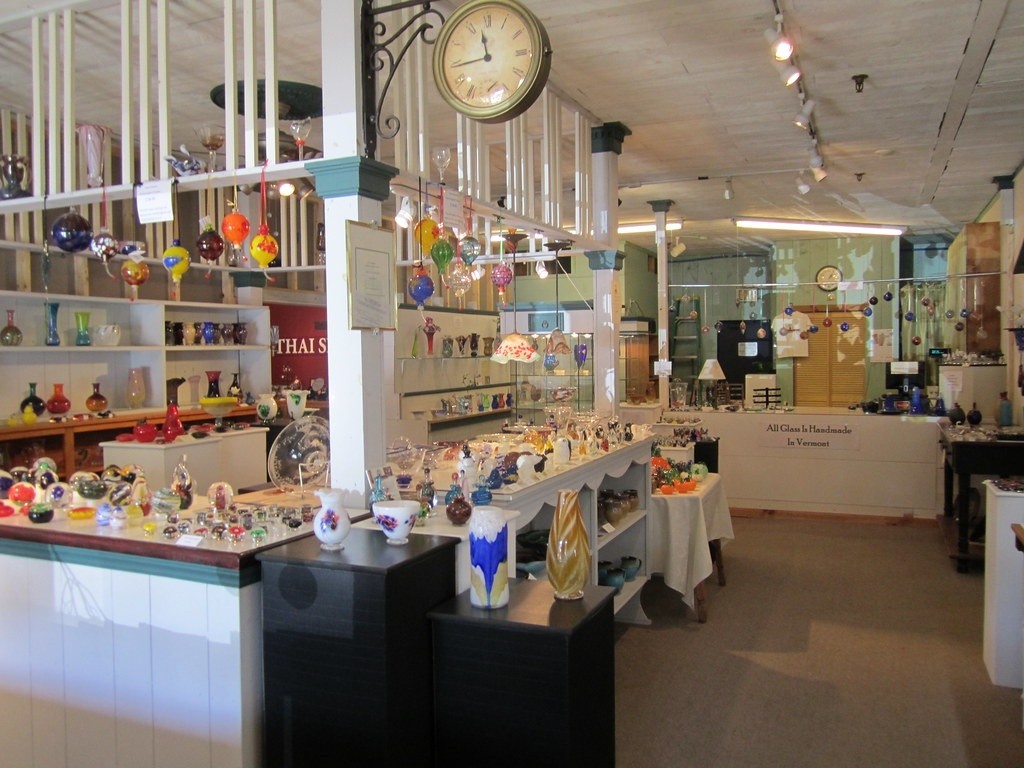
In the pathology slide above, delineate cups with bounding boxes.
[926,385,939,406]
[597,489,643,527]
[286,389,311,419]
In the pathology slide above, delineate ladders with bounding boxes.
[670,295,702,405]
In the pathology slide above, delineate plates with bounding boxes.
[267,415,330,495]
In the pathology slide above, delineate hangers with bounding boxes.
[785,306,793,314]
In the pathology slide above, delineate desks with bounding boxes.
[96,434,223,496]
[695,435,722,473]
[936,422,1024,572]
[657,440,697,464]
[212,425,272,493]
[650,472,736,625]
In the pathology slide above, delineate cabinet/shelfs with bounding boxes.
[941,219,1002,357]
[0,286,656,768]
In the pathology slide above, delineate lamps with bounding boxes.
[535,261,548,279]
[791,92,816,129]
[233,129,316,201]
[670,237,686,257]
[490,219,684,241]
[768,56,801,86]
[732,215,909,238]
[543,241,572,355]
[808,145,824,168]
[394,195,417,229]
[491,233,541,361]
[723,177,735,201]
[698,359,726,413]
[793,170,810,195]
[810,166,828,183]
[764,14,793,60]
[472,266,485,281]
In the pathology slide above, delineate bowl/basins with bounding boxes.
[134,424,157,442]
[371,500,422,544]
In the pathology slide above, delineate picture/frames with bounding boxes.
[345,218,400,336]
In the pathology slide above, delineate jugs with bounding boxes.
[597,555,643,593]
[312,487,352,551]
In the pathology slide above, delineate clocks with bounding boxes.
[815,266,843,292]
[433,0,553,125]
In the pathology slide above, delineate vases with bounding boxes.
[499,392,505,407]
[312,487,351,550]
[415,213,440,258]
[20,381,46,416]
[202,321,213,344]
[173,322,184,346]
[184,322,196,345]
[194,321,202,344]
[74,312,91,346]
[419,466,436,508]
[543,406,573,437]
[44,303,61,345]
[227,372,245,403]
[442,338,454,357]
[204,370,222,398]
[456,334,468,355]
[471,474,492,506]
[370,474,391,513]
[660,484,673,495]
[282,389,311,421]
[163,401,180,442]
[483,337,494,355]
[482,392,490,410]
[46,383,71,414]
[468,332,481,355]
[213,323,221,344]
[543,354,559,370]
[93,324,122,346]
[188,376,200,403]
[1,309,22,346]
[232,322,249,345]
[372,500,421,544]
[678,484,687,493]
[85,382,108,413]
[469,503,512,612]
[683,478,696,491]
[491,394,498,409]
[446,496,471,524]
[445,472,465,505]
[543,488,592,603]
[127,367,146,408]
[506,393,512,407]
[574,343,587,373]
[578,431,586,455]
[165,321,172,343]
[421,316,439,355]
[256,392,278,422]
[416,483,434,527]
[221,323,233,344]
[75,123,112,188]
[0,153,31,200]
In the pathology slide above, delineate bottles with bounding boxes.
[948,390,1010,426]
[165,318,247,345]
[545,488,589,600]
[227,372,243,399]
[86,383,107,411]
[911,382,919,403]
[440,331,494,356]
[469,505,511,609]
[46,383,71,414]
[205,370,222,398]
[127,366,146,408]
[74,312,91,346]
[0,308,23,346]
[369,477,389,517]
[163,403,185,441]
[476,392,514,411]
[22,405,36,424]
[256,393,277,419]
[43,302,61,345]
[96,325,121,346]
[410,444,493,526]
[20,382,45,417]
